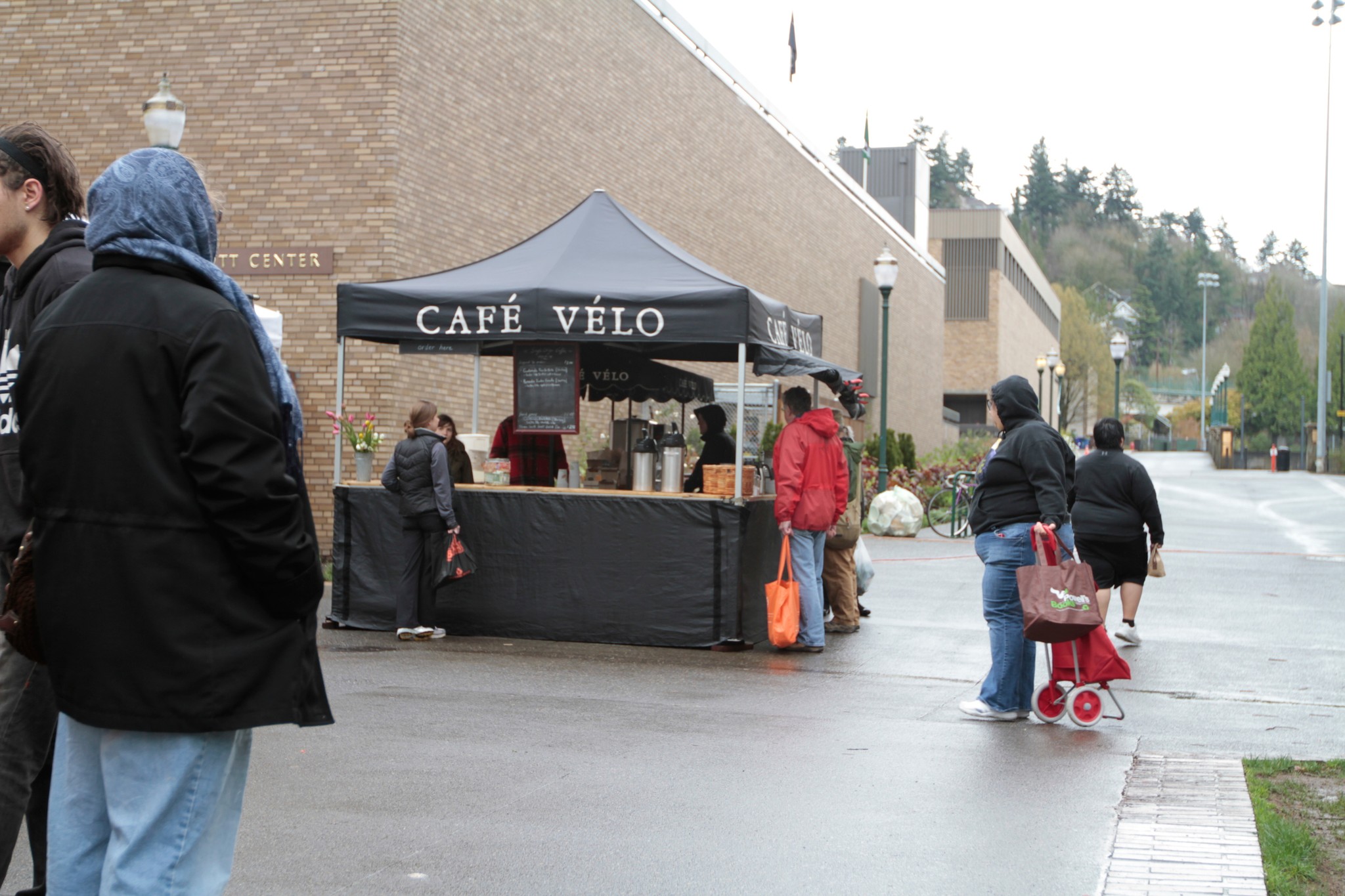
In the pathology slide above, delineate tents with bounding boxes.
[329,192,828,658]
[577,344,716,491]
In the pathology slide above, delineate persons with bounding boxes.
[0,121,96,896]
[682,404,736,494]
[1064,416,1166,646]
[487,415,572,489]
[381,399,464,643]
[805,424,872,620]
[9,141,336,896]
[432,412,474,486]
[827,407,865,634]
[959,374,1079,725]
[772,387,849,654]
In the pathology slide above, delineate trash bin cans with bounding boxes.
[1276,445,1290,471]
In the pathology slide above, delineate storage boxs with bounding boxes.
[582,449,622,490]
[701,463,756,496]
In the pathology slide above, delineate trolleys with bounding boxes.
[1027,524,1124,727]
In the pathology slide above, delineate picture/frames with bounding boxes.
[512,339,581,436]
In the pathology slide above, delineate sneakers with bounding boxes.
[859,607,871,616]
[430,626,445,640]
[958,697,1019,721]
[1114,621,1142,647]
[823,605,830,615]
[823,620,855,635]
[778,641,824,654]
[1016,708,1030,718]
[396,625,435,641]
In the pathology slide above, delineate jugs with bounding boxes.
[744,460,773,494]
[631,421,687,493]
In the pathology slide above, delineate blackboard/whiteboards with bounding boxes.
[512,340,580,435]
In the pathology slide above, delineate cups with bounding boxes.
[556,462,580,489]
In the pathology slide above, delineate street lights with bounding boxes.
[1035,350,1047,417]
[874,247,900,494]
[1197,271,1220,451]
[1215,375,1221,425]
[1110,331,1126,422]
[1310,0,1344,474]
[1222,363,1231,425]
[1046,347,1059,427]
[1219,369,1225,425]
[1209,382,1219,427]
[1054,358,1065,435]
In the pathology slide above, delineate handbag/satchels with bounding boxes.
[764,531,801,650]
[1146,542,1166,579]
[853,527,875,595]
[1014,522,1103,644]
[427,532,478,593]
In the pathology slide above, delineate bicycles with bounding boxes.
[926,472,979,538]
